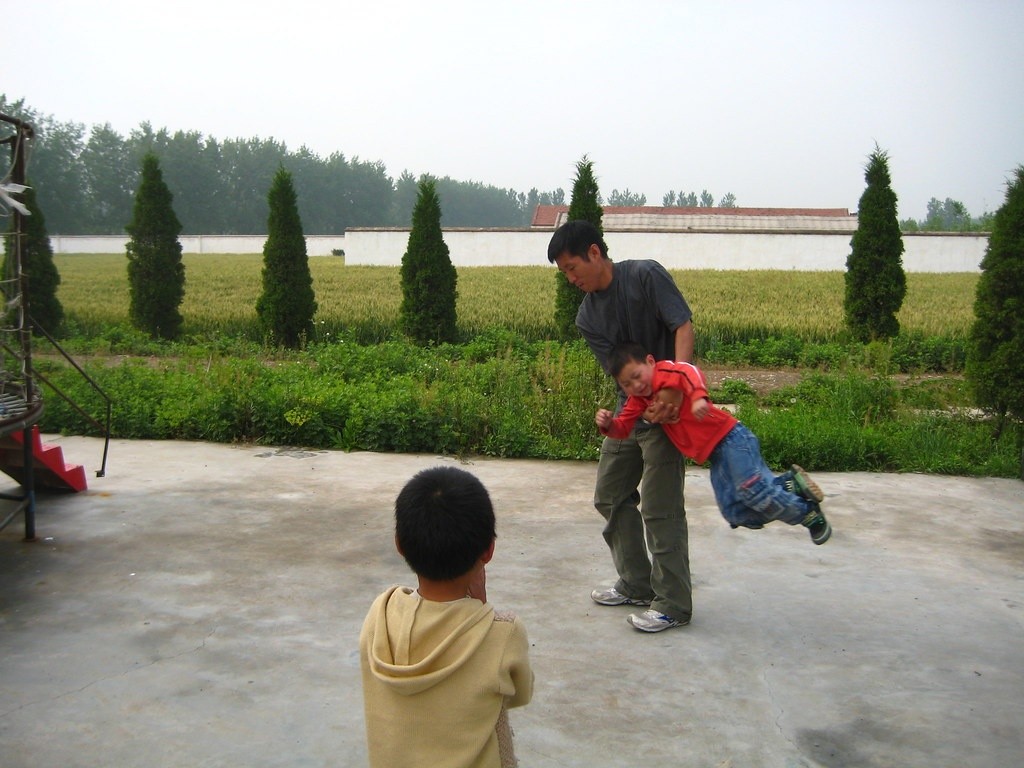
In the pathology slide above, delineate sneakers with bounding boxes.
[781,463,824,505]
[626,609,692,635]
[802,500,833,545]
[590,586,653,607]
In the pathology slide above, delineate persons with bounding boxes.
[596,341,831,545]
[358,466,534,768]
[548,220,693,632]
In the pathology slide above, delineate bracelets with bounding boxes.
[641,417,651,426]
